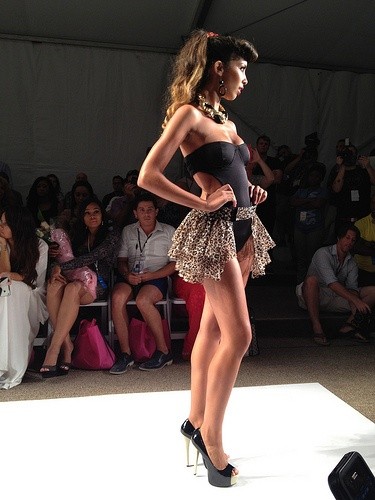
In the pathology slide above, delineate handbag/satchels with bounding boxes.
[72,318,116,370]
[245,306,259,358]
[128,317,172,363]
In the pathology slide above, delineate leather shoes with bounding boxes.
[138,350,173,371]
[110,352,134,374]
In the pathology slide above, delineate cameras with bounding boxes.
[352,309,375,335]
[339,149,356,166]
[127,176,138,188]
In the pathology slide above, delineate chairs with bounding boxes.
[30,262,188,346]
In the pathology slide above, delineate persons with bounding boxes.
[0,133,375,389]
[138,30,276,487]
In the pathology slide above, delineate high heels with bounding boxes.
[56,361,71,376]
[181,418,207,469]
[41,365,56,378]
[192,428,239,487]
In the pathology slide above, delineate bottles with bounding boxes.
[131,264,144,276]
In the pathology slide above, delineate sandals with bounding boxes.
[313,332,328,345]
[340,324,367,343]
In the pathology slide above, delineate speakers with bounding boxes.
[328,452,375,500]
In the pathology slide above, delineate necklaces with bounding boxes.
[194,92,229,125]
